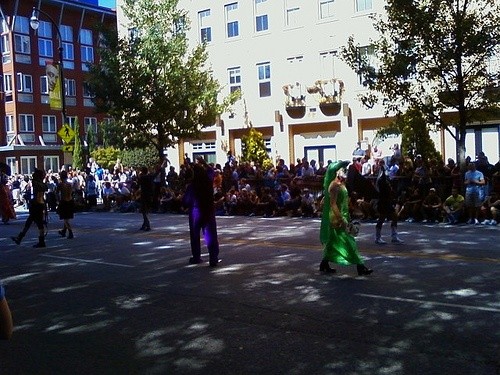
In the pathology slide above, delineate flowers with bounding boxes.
[282,81,306,106]
[307,80,344,103]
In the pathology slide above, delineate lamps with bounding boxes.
[343,103,352,127]
[274,111,284,132]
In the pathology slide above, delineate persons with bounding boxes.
[0,141,500,226]
[182,155,219,267]
[11,167,48,247]
[319,159,373,276]
[374,166,404,243]
[46,64,59,91]
[55,170,74,239]
[138,168,154,231]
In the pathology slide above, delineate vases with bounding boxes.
[286,106,306,118]
[319,102,341,117]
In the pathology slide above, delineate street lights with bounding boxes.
[30,6,66,124]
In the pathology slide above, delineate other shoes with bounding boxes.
[209,259,218,266]
[140,224,151,232]
[189,256,202,263]
[33,235,46,248]
[391,237,405,244]
[403,216,498,226]
[67,231,74,240]
[11,232,25,244]
[58,229,66,237]
[320,262,337,273]
[375,237,386,244]
[357,265,372,276]
